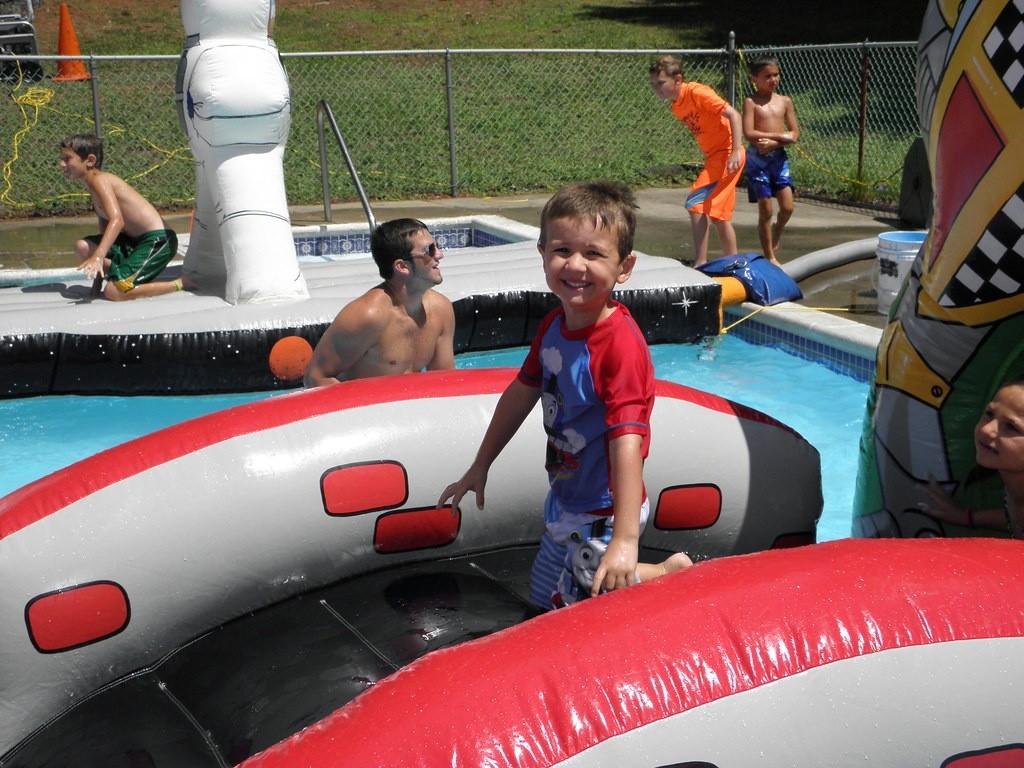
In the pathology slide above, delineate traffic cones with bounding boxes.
[52,2,93,81]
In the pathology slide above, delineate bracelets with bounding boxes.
[968,506,979,530]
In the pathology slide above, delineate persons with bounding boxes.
[437,175,693,609]
[303,217,457,388]
[742,56,801,269]
[649,53,747,269]
[914,376,1024,539]
[58,133,199,303]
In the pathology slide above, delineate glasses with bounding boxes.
[403,242,438,261]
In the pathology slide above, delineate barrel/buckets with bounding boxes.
[871,230,932,317]
